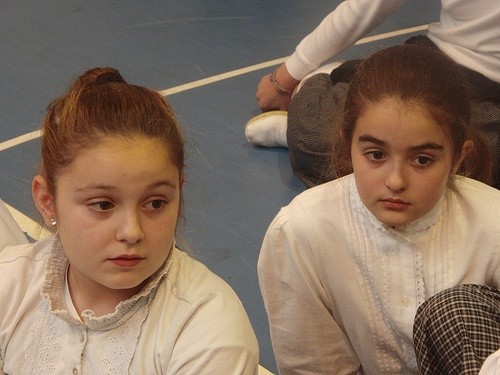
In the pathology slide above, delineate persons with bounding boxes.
[254,35,499,374]
[244,1,498,191]
[0,66,262,375]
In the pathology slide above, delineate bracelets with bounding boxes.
[268,64,295,98]
